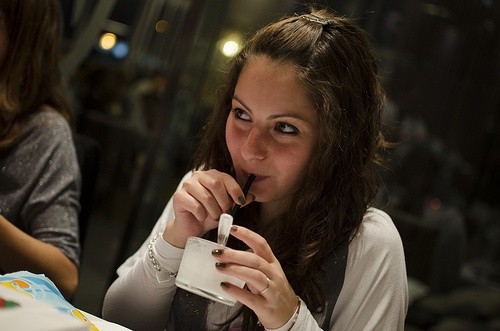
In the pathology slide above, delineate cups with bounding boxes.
[175,236,246,306]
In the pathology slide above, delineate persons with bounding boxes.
[99,12,408,331]
[0,0,80,303]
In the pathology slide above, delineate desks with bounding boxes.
[69,108,171,315]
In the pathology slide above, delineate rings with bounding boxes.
[256,278,272,295]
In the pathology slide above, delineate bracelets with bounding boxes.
[147,233,178,276]
[287,295,301,331]
